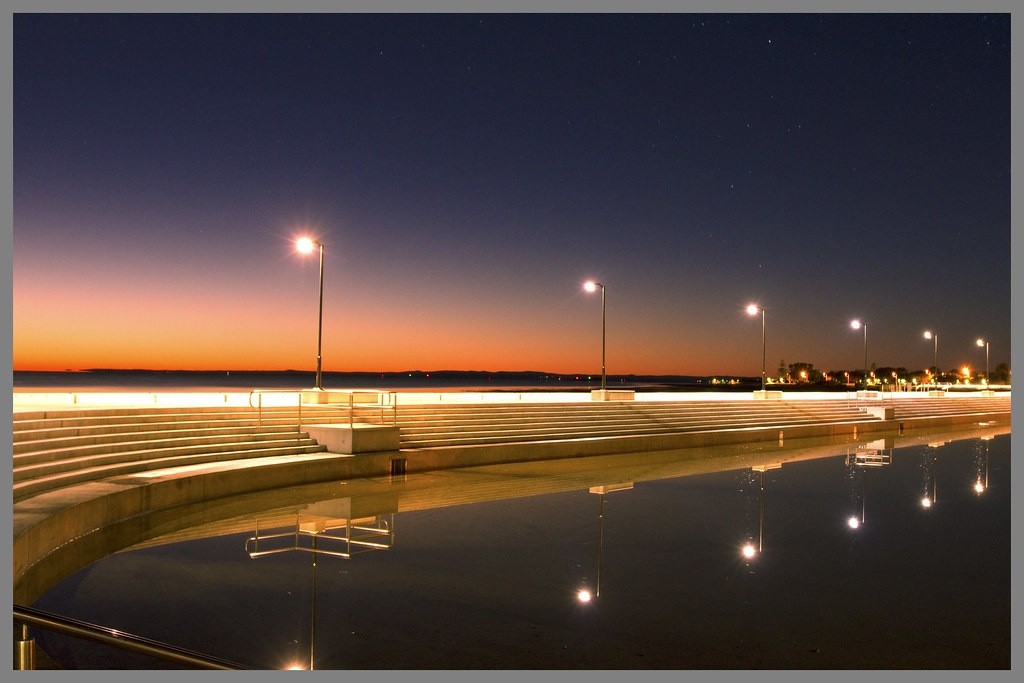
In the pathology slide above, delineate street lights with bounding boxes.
[925,330,938,391]
[287,536,318,669]
[584,278,608,390]
[850,319,868,391]
[920,447,938,508]
[740,473,766,559]
[747,303,767,390]
[296,236,324,391]
[974,440,990,493]
[847,466,866,528]
[977,339,991,391]
[578,494,605,603]
